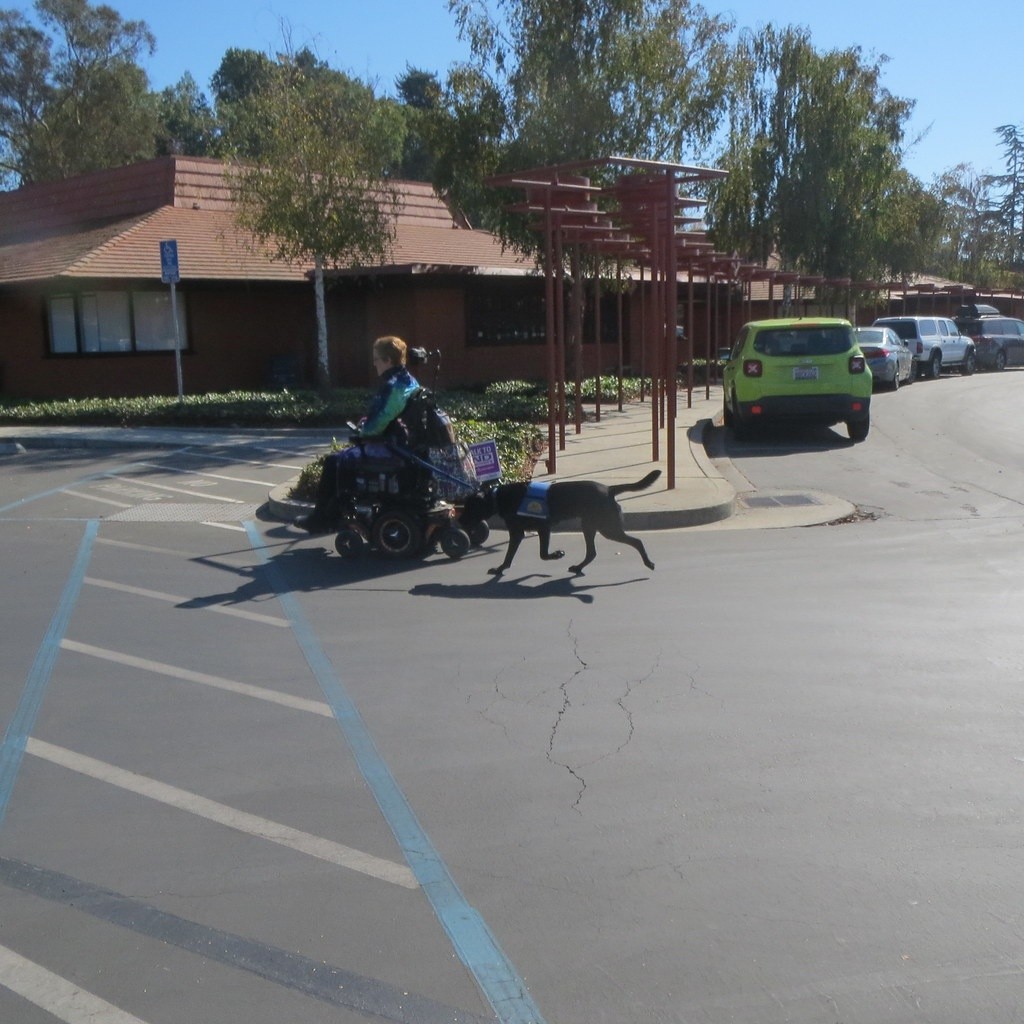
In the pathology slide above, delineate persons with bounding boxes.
[293,336,420,533]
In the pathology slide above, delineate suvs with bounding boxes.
[871,316,976,379]
[952,304,1024,371]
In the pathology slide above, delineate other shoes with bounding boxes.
[294,513,328,531]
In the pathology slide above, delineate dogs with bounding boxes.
[458,470,662,572]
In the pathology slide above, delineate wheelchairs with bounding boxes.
[336,388,489,559]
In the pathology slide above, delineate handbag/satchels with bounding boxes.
[429,438,483,502]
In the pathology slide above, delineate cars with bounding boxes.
[722,317,873,439]
[852,327,917,390]
